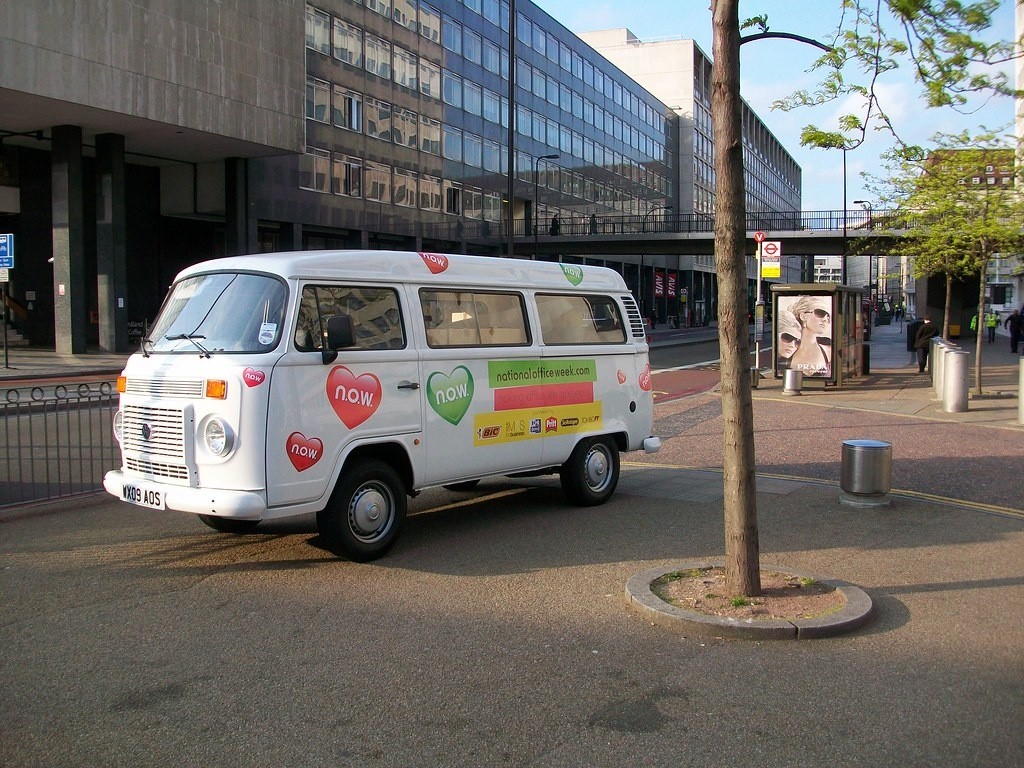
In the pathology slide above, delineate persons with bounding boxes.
[642,309,657,329]
[455,217,490,240]
[970,309,1023,353]
[914,315,939,372]
[749,310,771,326]
[778,296,832,378]
[589,215,598,235]
[889,307,904,323]
[552,215,559,235]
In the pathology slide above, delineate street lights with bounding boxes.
[787,257,795,284]
[535,154,560,261]
[895,265,901,306]
[876,257,883,301]
[642,206,673,233]
[854,201,871,305]
[822,143,847,284]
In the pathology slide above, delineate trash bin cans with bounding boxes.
[1018,355,1024,430]
[928,336,971,413]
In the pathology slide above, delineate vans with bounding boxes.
[103,249,661,563]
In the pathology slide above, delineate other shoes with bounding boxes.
[919,368,924,371]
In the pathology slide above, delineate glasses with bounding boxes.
[779,333,802,348]
[804,309,831,324]
